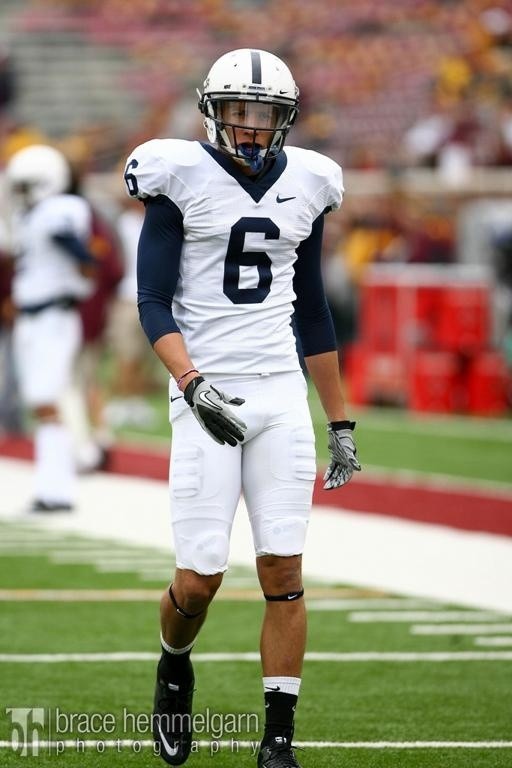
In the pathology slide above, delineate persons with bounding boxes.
[2,1,511,513]
[121,43,358,767]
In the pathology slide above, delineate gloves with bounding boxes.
[183,376,247,447]
[321,420,360,491]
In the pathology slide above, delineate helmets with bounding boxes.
[202,46,299,171]
[9,145,72,203]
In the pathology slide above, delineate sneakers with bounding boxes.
[152,674,195,765]
[258,741,301,768]
